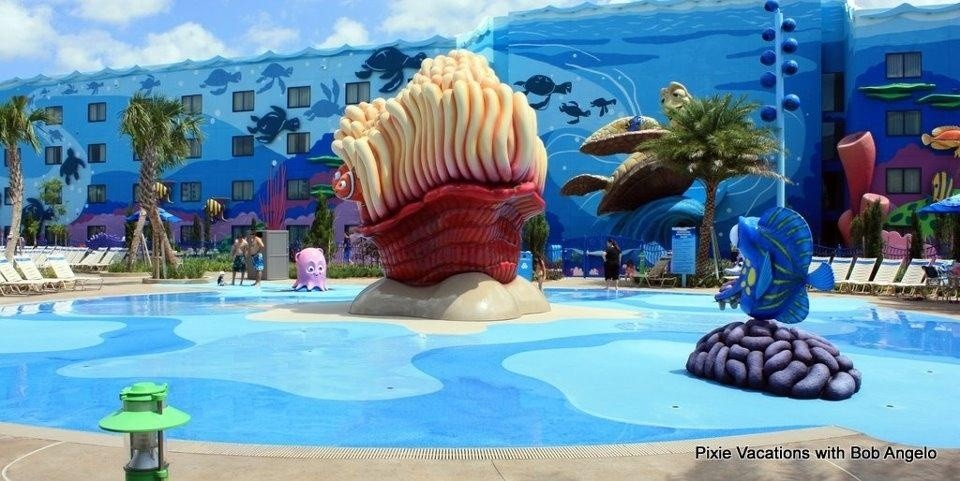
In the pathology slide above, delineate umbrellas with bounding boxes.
[121,207,184,257]
[916,194,960,217]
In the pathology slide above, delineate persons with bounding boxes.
[217,270,228,286]
[340,233,356,269]
[601,239,623,292]
[247,232,266,287]
[532,252,548,292]
[229,235,250,287]
[623,259,636,287]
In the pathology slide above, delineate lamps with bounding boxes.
[100,381,191,480]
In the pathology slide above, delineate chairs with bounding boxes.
[618,256,677,288]
[808,256,956,302]
[0,245,129,296]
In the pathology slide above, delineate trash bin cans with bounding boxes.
[247,230,290,281]
[517,251,532,282]
